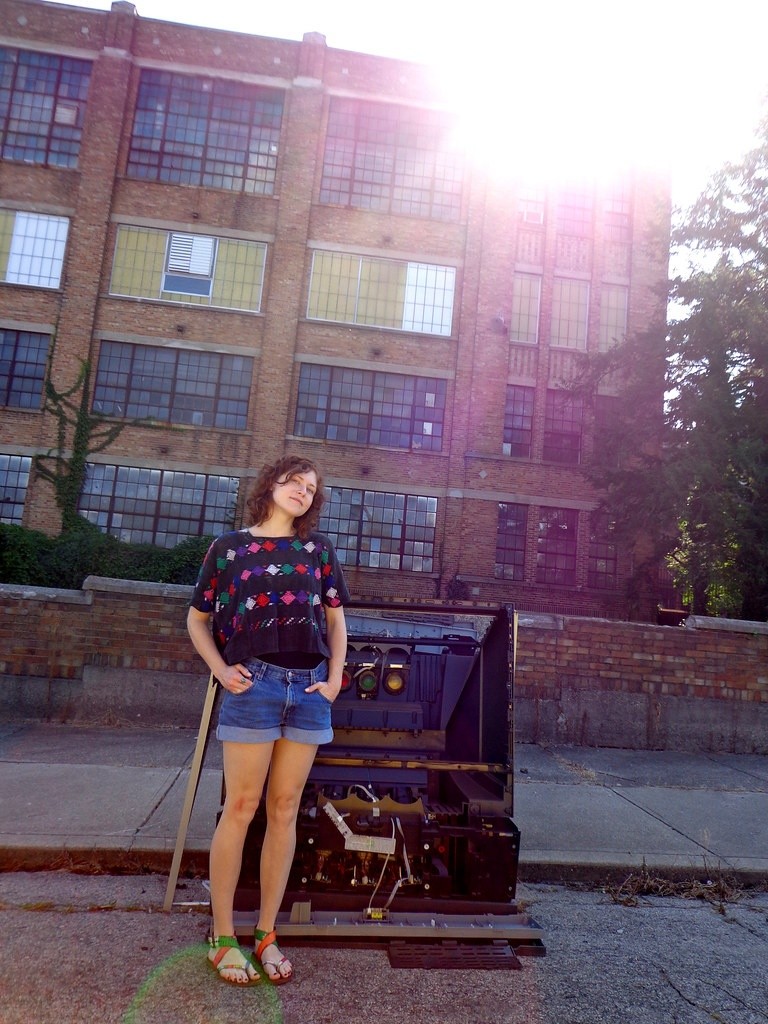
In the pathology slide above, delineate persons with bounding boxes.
[185,454,351,987]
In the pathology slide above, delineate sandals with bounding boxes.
[252,925,293,986]
[206,932,263,988]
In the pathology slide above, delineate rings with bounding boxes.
[240,677,245,684]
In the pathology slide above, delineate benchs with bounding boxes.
[657,602,692,625]
